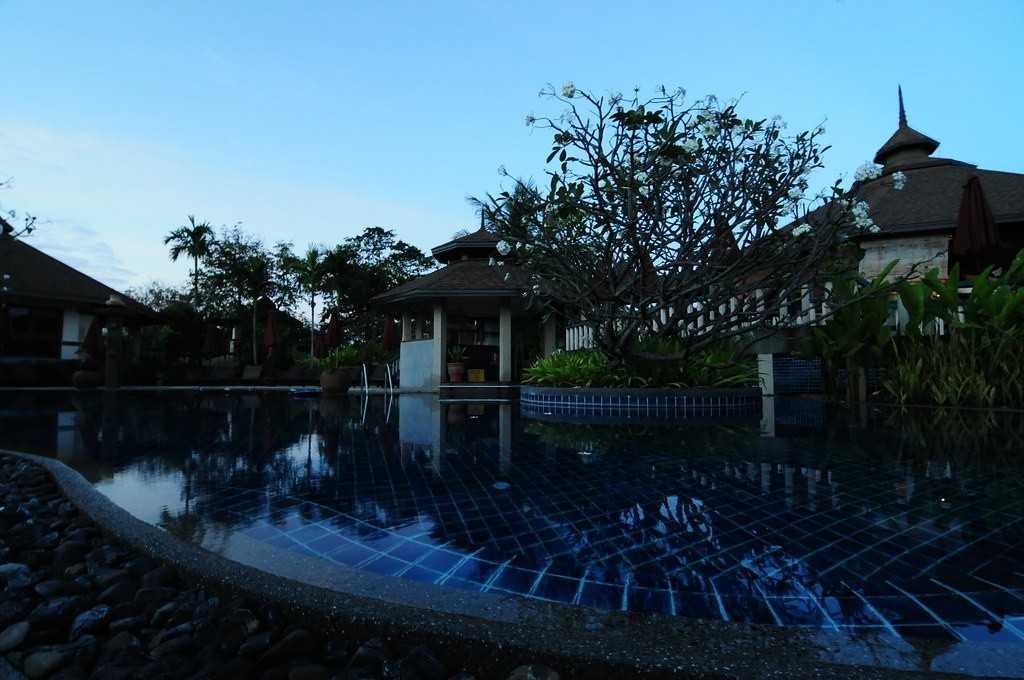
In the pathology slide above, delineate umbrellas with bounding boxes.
[324,310,340,346]
[709,214,742,280]
[263,311,284,348]
[202,324,218,352]
[585,260,609,303]
[381,315,400,350]
[950,175,1000,252]
[634,251,657,297]
[312,330,330,359]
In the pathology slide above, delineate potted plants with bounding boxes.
[447,404,468,447]
[447,344,472,383]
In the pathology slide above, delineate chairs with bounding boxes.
[194,364,391,389]
[225,391,264,418]
